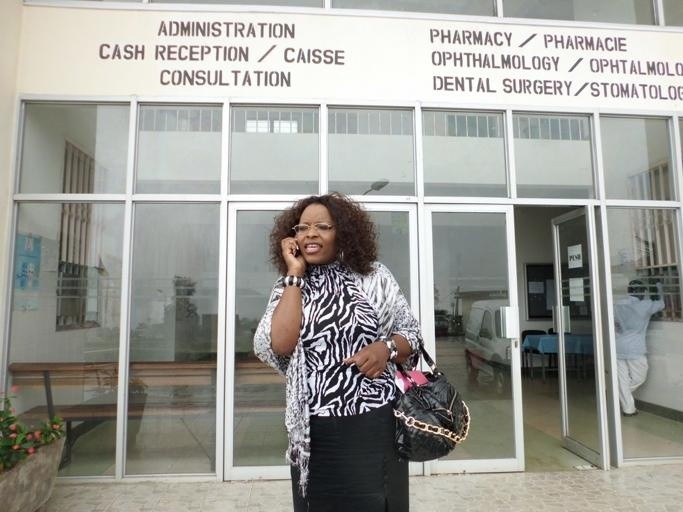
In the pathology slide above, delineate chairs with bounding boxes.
[523,328,577,379]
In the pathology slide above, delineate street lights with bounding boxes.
[362,179,389,195]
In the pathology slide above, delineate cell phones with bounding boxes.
[292,242,300,257]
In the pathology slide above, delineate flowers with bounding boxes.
[0,386,64,476]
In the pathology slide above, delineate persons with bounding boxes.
[613,279,667,415]
[253,191,425,512]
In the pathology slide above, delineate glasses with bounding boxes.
[291,223,336,234]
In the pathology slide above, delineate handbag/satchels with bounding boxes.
[393,344,471,462]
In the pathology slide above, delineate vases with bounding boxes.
[0,436,68,512]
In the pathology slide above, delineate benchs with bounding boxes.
[10,359,288,472]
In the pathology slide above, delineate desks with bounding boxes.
[524,334,593,384]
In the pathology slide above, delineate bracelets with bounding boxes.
[282,275,306,289]
[379,335,399,359]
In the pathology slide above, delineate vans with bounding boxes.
[464,300,518,396]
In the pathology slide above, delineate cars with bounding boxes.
[435,314,449,334]
